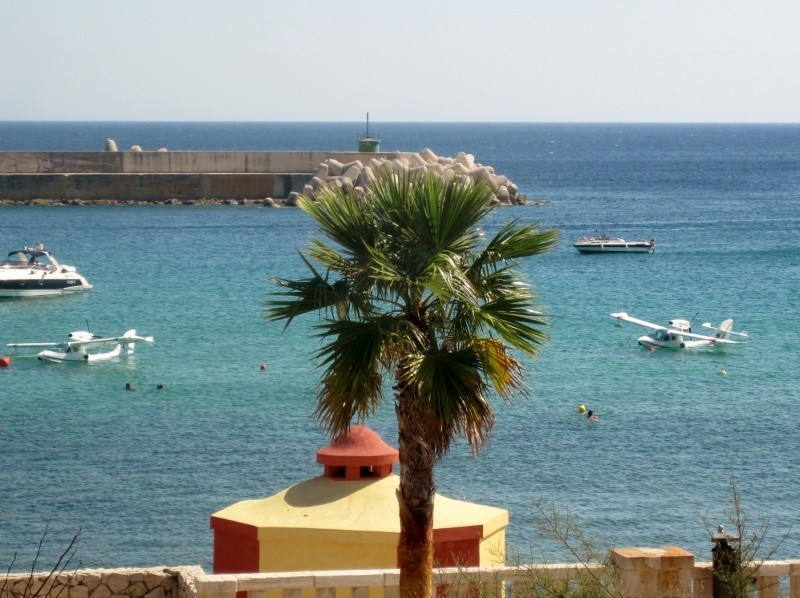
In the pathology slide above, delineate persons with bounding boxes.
[586,411,599,422]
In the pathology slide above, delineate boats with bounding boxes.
[0,240,93,298]
[5,329,154,365]
[610,312,748,351]
[573,228,655,255]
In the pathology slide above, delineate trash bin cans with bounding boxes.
[711,534,742,598]
